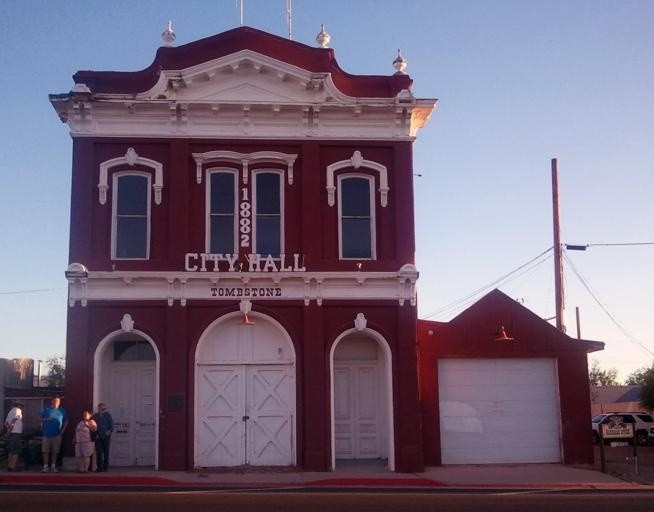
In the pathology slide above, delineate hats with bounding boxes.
[14,400,24,407]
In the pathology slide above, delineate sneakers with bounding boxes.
[40,467,48,473]
[49,467,58,473]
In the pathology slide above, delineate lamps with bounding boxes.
[237,300,257,326]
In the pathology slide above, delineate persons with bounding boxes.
[37,394,69,473]
[89,403,114,472]
[70,406,97,473]
[2,399,24,472]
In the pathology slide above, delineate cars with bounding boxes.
[592,411,654,445]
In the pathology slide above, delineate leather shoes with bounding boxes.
[77,467,108,472]
[10,468,21,472]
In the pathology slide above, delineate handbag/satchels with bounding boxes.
[90,430,100,441]
